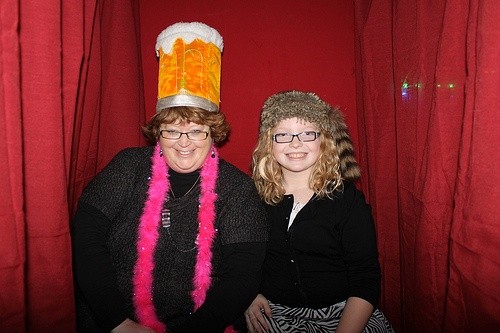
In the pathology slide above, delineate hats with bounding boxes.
[260,90,361,180]
[155,22,224,112]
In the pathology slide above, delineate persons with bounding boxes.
[240,89,393,333]
[71,21,271,333]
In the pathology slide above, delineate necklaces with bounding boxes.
[167,172,202,197]
[166,204,195,255]
[133,140,219,332]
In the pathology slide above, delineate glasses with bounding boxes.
[159,129,209,141]
[272,131,321,143]
